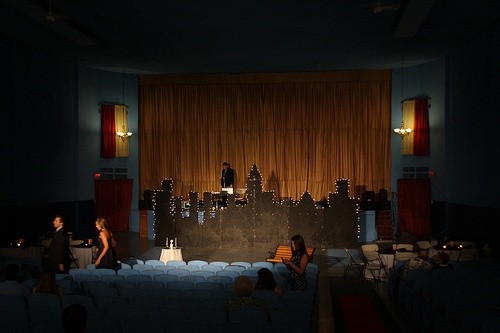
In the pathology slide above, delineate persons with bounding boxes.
[61,304,90,333]
[255,268,282,294]
[87,217,118,270]
[224,275,270,320]
[221,162,235,188]
[397,250,433,280]
[32,217,71,302]
[432,251,463,278]
[280,234,310,291]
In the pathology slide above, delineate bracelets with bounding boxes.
[292,265,296,269]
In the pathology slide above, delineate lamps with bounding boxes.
[394,122,411,139]
[117,126,132,142]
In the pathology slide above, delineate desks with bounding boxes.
[0,247,93,268]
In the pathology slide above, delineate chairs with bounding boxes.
[0,236,499,332]
